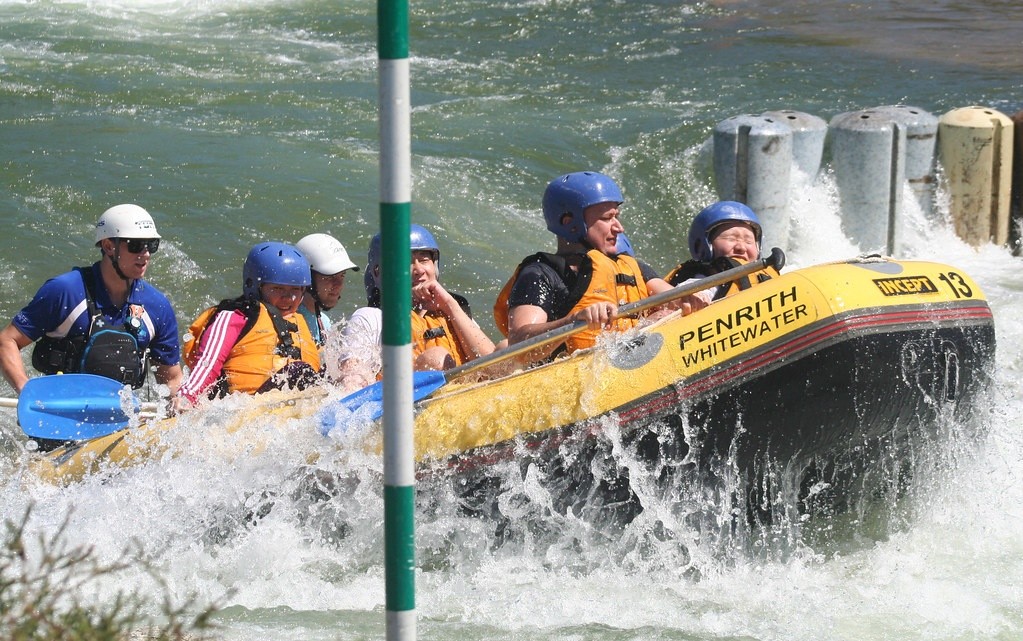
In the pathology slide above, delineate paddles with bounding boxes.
[315,247,786,437]
[0,397,18,407]
[17,373,166,440]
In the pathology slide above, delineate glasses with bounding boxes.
[118,238,160,253]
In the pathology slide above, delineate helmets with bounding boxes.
[95,204,162,247]
[242,242,311,301]
[615,232,635,257]
[689,200,763,263]
[368,224,440,290]
[295,233,360,276]
[543,170,624,242]
[364,264,378,302]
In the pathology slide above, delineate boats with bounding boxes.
[18,256,997,538]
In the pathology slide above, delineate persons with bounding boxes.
[0,205,182,454]
[337,225,496,392]
[167,242,321,416]
[494,171,782,366]
[296,234,361,342]
